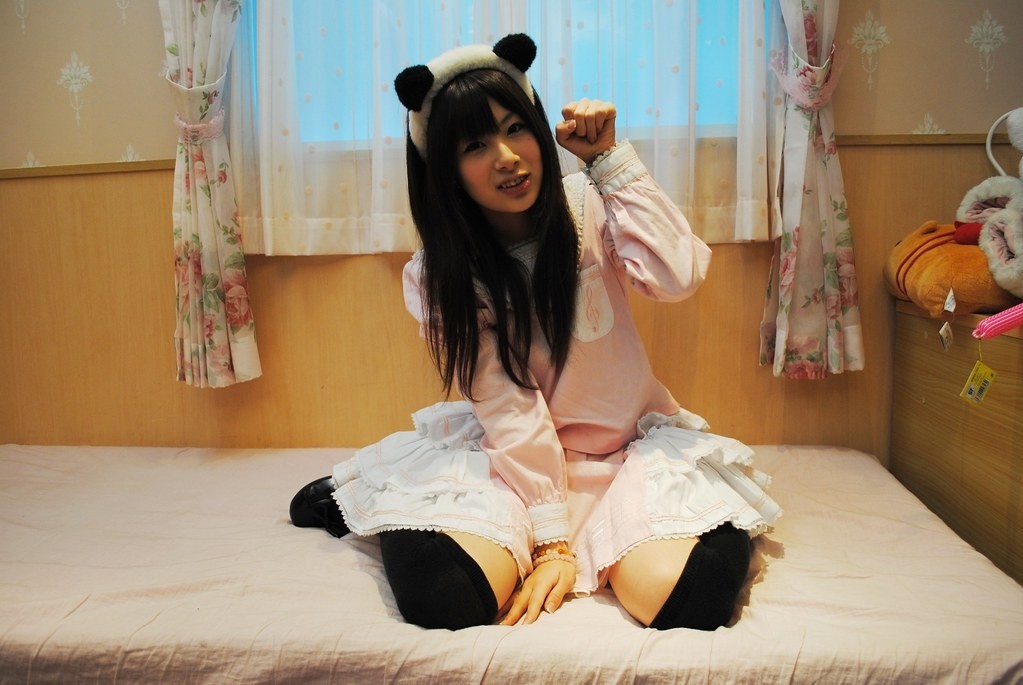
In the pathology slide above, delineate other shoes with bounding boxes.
[290,475,353,538]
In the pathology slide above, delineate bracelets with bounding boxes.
[531,548,579,567]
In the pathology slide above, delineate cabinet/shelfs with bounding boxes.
[889,301,1023,584]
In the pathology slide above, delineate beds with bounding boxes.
[0,447,1023,685]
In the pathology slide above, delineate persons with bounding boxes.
[290,31,785,632]
